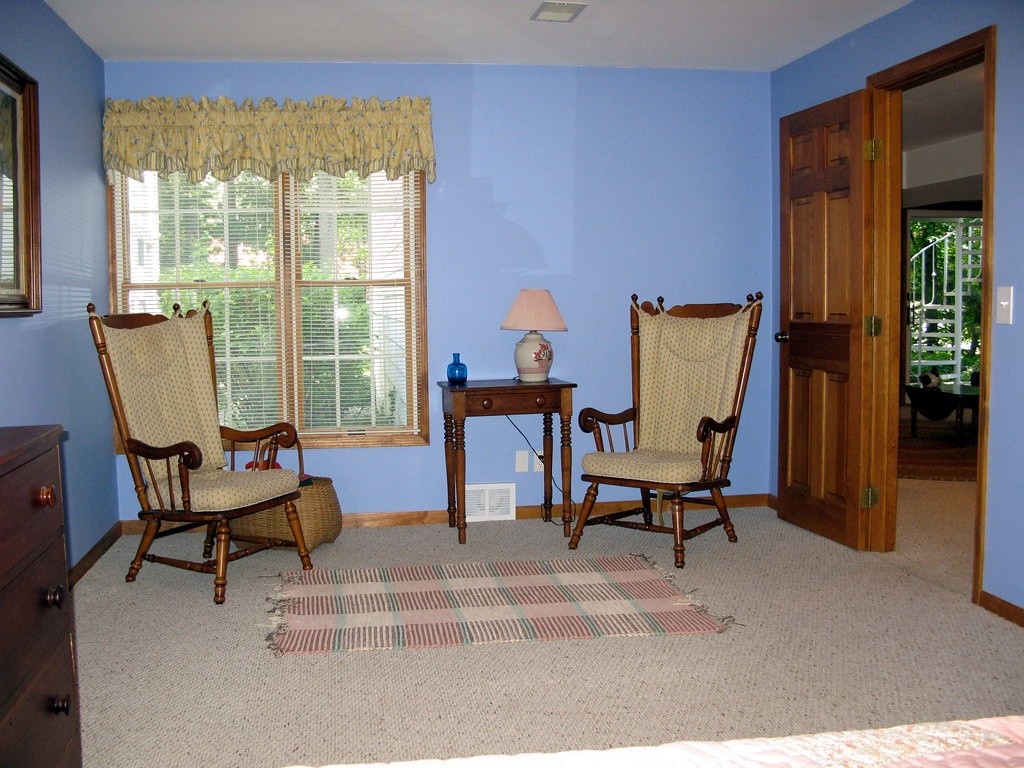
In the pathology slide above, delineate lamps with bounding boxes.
[500,289,568,383]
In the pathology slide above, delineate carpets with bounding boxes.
[255,552,746,649]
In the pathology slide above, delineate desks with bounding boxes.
[905,382,980,442]
[437,377,578,544]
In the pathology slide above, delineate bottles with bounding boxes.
[447,353,467,385]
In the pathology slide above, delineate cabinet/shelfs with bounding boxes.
[0,424,83,768]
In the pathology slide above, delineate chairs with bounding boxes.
[87,301,312,605]
[569,291,763,568]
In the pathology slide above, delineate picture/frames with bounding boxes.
[1,53,44,316]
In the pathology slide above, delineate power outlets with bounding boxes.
[534,451,544,473]
